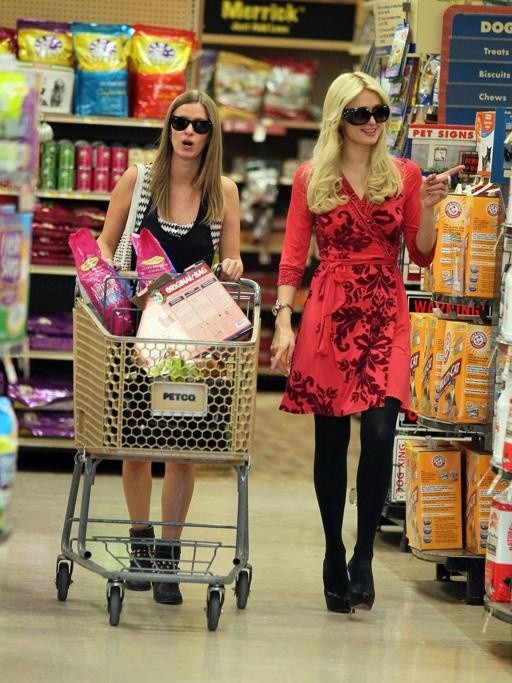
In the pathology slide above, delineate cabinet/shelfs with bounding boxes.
[1,112,165,450]
[196,35,354,377]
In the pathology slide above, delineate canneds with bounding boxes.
[39,139,159,193]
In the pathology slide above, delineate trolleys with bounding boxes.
[55,267,262,632]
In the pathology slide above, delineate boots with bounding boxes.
[125,525,155,591]
[152,538,183,604]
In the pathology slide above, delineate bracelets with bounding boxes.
[270,297,293,317]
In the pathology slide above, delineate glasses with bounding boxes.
[342,103,390,125]
[169,115,212,135]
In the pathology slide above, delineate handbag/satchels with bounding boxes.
[113,164,147,273]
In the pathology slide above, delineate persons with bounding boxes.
[264,69,467,614]
[93,87,245,606]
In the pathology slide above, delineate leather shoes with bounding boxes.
[348,553,375,611]
[323,557,352,614]
